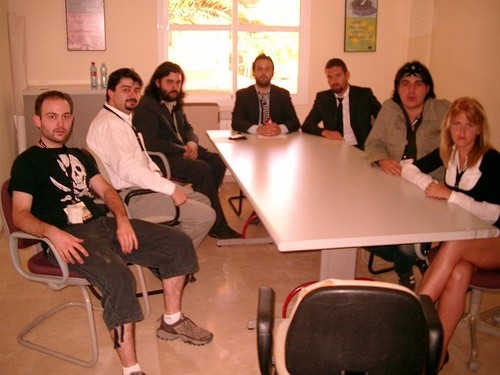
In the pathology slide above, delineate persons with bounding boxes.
[7,91,214,375]
[132,62,243,240]
[401,97,500,374]
[86,68,216,284]
[364,61,452,292]
[301,59,382,151]
[231,53,301,225]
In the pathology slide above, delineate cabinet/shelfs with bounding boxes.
[23,85,106,149]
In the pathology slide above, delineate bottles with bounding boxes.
[100,63,108,88]
[90,62,97,88]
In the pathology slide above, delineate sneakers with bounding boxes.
[399,267,416,292]
[122,371,146,375]
[156,314,214,347]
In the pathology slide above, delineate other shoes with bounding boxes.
[248,213,260,225]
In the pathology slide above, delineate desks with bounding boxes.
[206,128,500,283]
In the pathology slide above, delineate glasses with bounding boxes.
[399,82,427,89]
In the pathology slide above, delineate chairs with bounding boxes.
[257,278,443,375]
[414,241,500,371]
[88,155,189,300]
[0,179,151,368]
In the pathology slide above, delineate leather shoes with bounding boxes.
[147,266,162,280]
[208,226,241,239]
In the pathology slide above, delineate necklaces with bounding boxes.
[411,113,420,126]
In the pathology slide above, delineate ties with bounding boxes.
[337,97,344,139]
